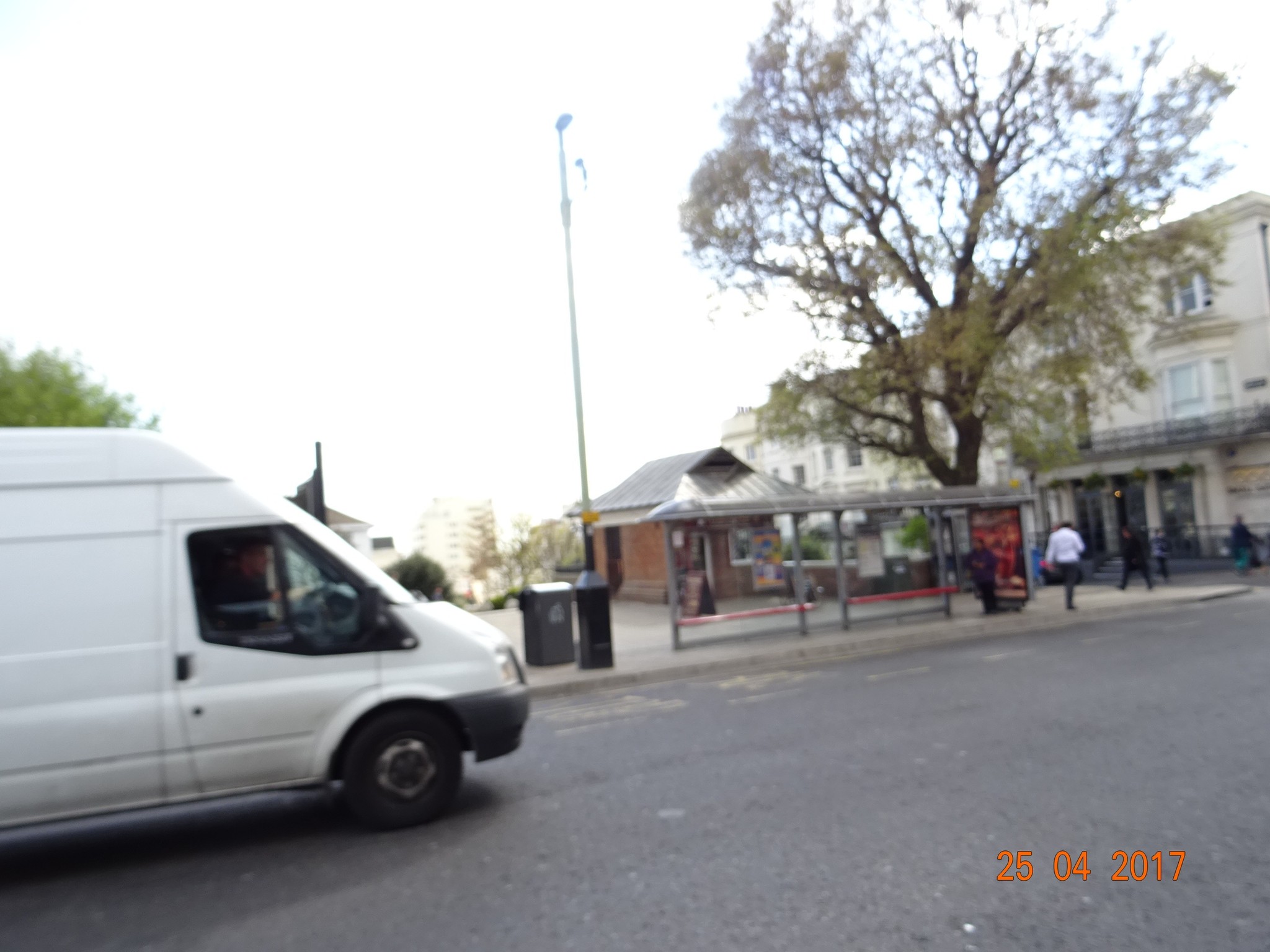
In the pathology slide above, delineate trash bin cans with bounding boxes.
[883,555,914,592]
[523,581,575,666]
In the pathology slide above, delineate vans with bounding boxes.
[0,427,539,862]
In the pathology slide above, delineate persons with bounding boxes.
[204,542,283,631]
[964,537,998,614]
[1229,515,1264,576]
[1044,523,1085,610]
[1149,529,1172,582]
[1116,525,1153,591]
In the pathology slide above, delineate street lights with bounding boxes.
[554,112,617,670]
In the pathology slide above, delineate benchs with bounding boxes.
[844,586,962,606]
[674,601,815,628]
